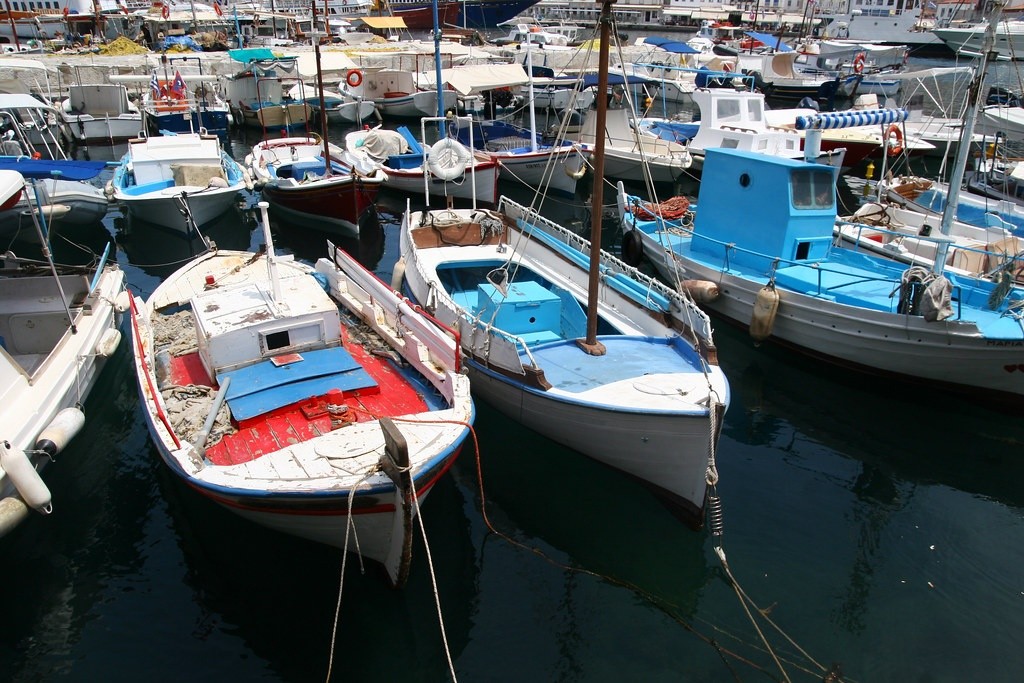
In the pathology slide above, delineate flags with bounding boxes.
[928,2,937,9]
[172,71,186,92]
[149,70,160,97]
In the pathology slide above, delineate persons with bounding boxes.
[790,173,828,206]
[140,21,152,50]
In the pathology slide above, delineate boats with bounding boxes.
[120,195,481,585]
[0,169,141,551]
[393,0,733,518]
[613,109,1024,396]
[0,1,1024,245]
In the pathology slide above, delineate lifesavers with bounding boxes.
[214,3,222,16]
[853,53,864,72]
[254,14,259,21]
[884,123,903,156]
[343,0,347,5]
[428,137,468,181]
[160,81,182,104]
[620,230,643,266]
[162,6,170,19]
[347,69,362,87]
